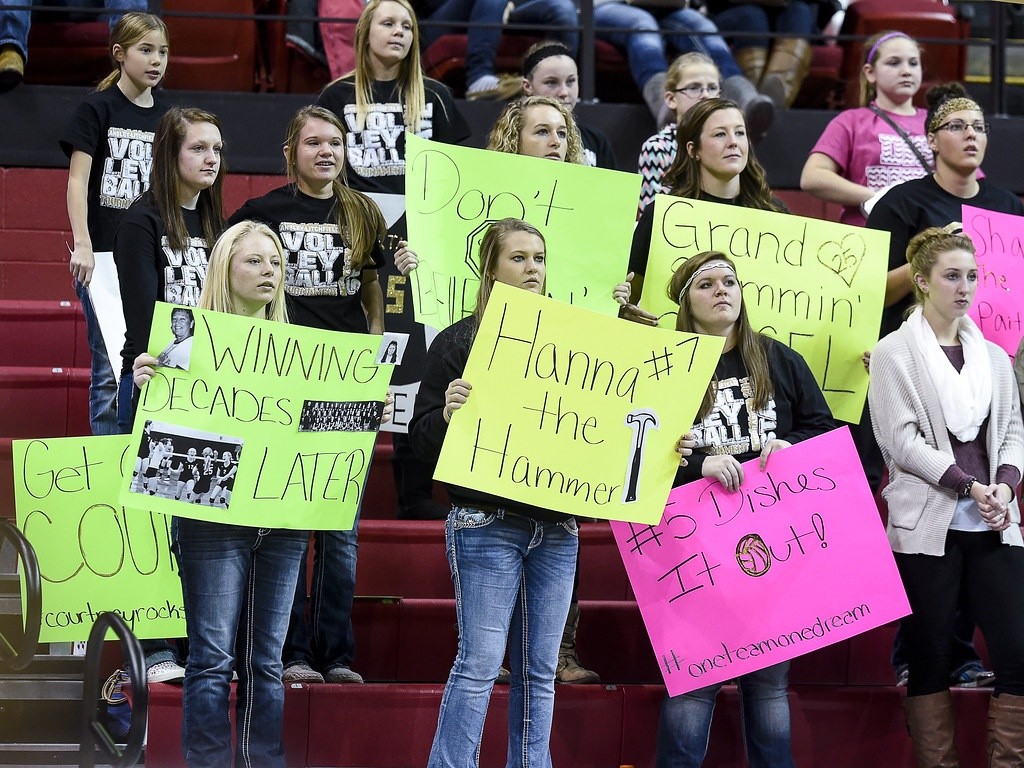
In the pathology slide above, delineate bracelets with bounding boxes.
[964,477,977,497]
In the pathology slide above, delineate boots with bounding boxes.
[761,38,811,107]
[644,72,676,131]
[722,75,775,140]
[737,46,769,87]
[987,693,1024,768]
[904,689,960,768]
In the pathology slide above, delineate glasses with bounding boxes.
[932,120,990,135]
[673,86,722,99]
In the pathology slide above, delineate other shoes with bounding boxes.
[325,668,364,683]
[466,76,525,103]
[147,661,186,684]
[282,665,326,683]
[0,45,25,77]
[956,668,997,688]
[102,668,130,743]
[555,656,600,685]
[496,666,511,682]
[895,671,911,686]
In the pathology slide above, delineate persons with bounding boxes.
[132,221,393,767]
[0,0,149,87]
[523,38,618,173]
[301,402,381,431]
[156,307,194,369]
[131,420,241,507]
[394,96,637,685]
[422,0,1024,768]
[636,46,750,215]
[117,106,243,682]
[59,12,171,435]
[319,0,473,193]
[377,340,401,365]
[223,103,387,684]
[792,20,986,246]
